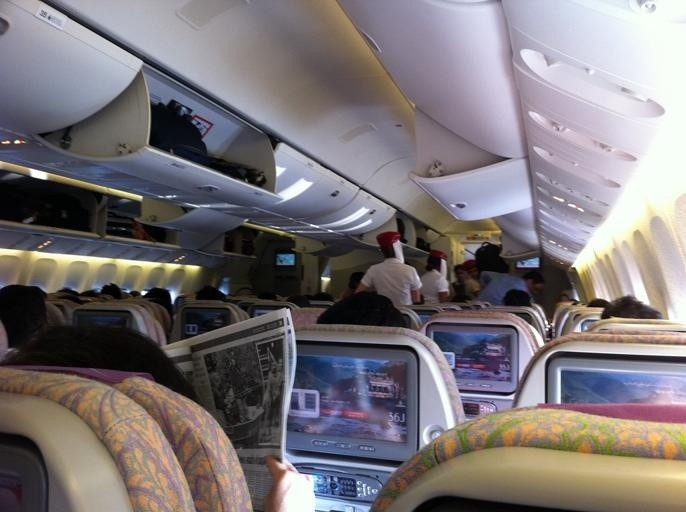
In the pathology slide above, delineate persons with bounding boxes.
[1,272,665,348]
[354,232,423,307]
[0,326,317,511]
[421,249,454,305]
[449,261,480,302]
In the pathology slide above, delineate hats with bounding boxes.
[461,260,476,271]
[429,248,447,260]
[376,231,401,248]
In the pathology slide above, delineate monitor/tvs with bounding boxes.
[77,315,127,332]
[274,252,296,267]
[515,257,541,270]
[287,355,408,444]
[433,330,511,383]
[181,310,229,337]
[562,369,686,405]
